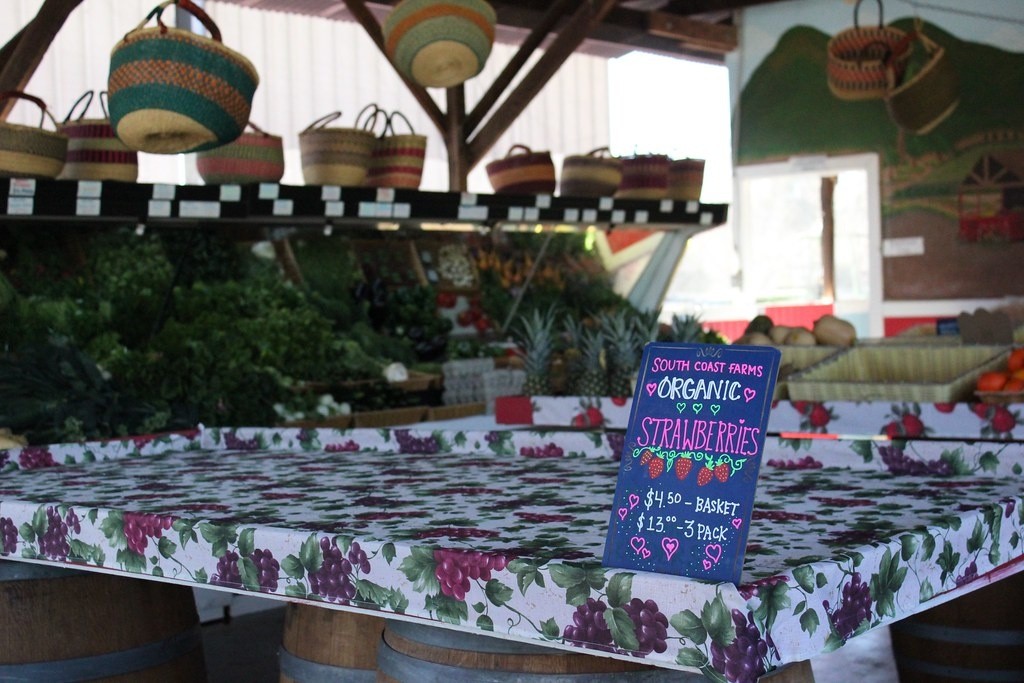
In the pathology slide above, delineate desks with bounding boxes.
[0,424,1024,683]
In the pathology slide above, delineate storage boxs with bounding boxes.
[496,344,1012,423]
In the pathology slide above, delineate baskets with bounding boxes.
[107,0,260,152]
[618,143,672,199]
[0,92,68,178]
[560,147,623,199]
[58,91,138,182]
[486,146,556,196]
[365,110,427,190]
[282,360,441,401]
[757,322,1024,405]
[884,12,966,136]
[382,0,497,88]
[196,120,284,184]
[826,0,914,102]
[299,104,377,184]
[670,158,705,200]
